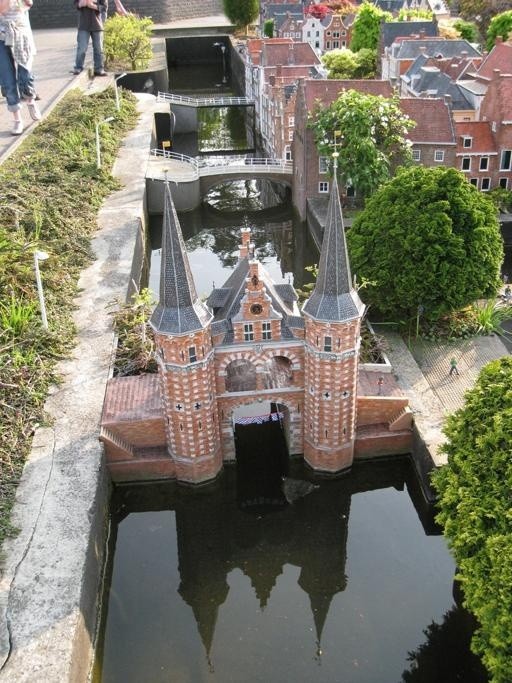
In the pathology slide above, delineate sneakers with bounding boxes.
[94,71,108,76]
[27,102,41,120]
[11,120,23,134]
[74,68,82,74]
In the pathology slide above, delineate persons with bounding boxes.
[377,376,384,394]
[71,0,108,76]
[449,355,460,376]
[0,0,42,136]
[98,0,128,22]
